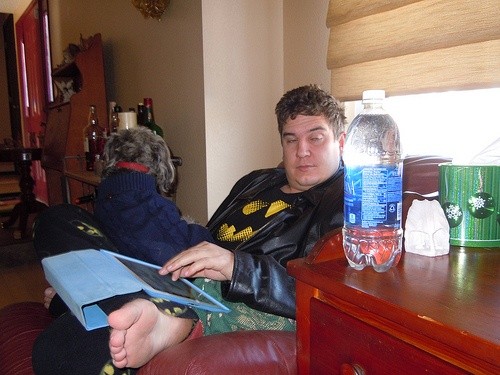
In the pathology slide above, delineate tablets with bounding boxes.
[100,248,231,313]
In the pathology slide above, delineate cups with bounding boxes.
[439,164,500,247]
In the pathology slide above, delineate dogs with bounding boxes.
[93,125,215,266]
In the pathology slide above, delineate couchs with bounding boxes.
[0,157,452,375]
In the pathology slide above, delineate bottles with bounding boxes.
[83,98,163,171]
[342,90,403,272]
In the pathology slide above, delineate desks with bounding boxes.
[0,147,49,239]
[286,227,500,375]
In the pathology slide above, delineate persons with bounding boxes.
[29,84,348,375]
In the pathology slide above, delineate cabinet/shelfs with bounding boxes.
[40,32,110,214]
[64,169,100,217]
